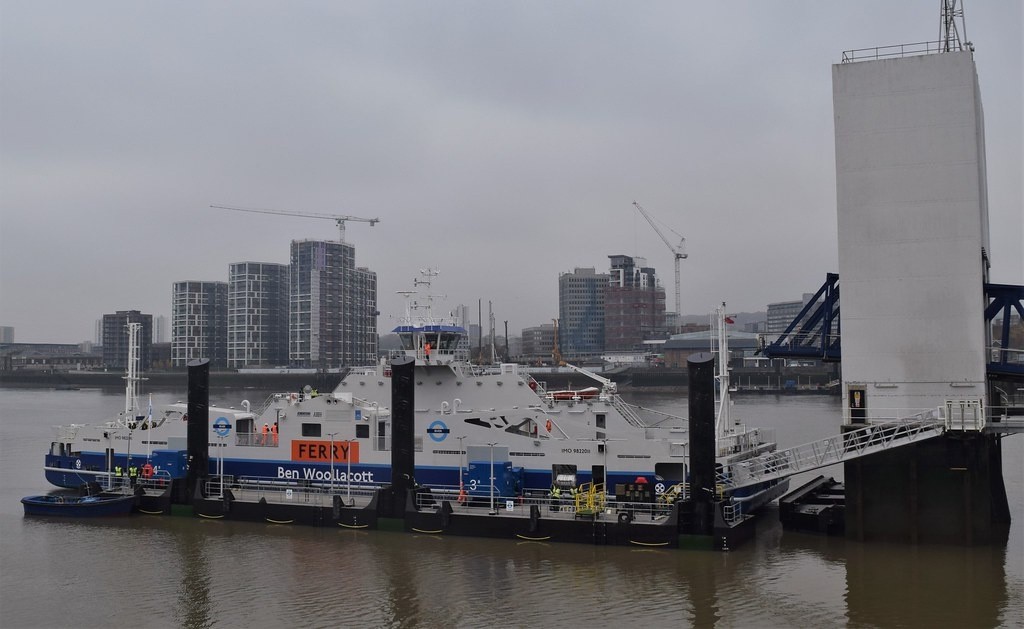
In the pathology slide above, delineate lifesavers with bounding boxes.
[142,465,154,478]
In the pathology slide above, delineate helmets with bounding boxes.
[141,464,144,466]
[131,463,134,465]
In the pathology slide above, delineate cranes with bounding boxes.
[632,200,688,335]
[209,205,380,244]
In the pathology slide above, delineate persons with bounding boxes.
[296,385,319,404]
[113,462,123,489]
[545,418,552,433]
[260,423,270,446]
[424,342,430,361]
[271,422,278,446]
[550,479,584,514]
[127,462,138,490]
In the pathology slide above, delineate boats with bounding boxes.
[43,264,793,523]
[20,494,140,517]
